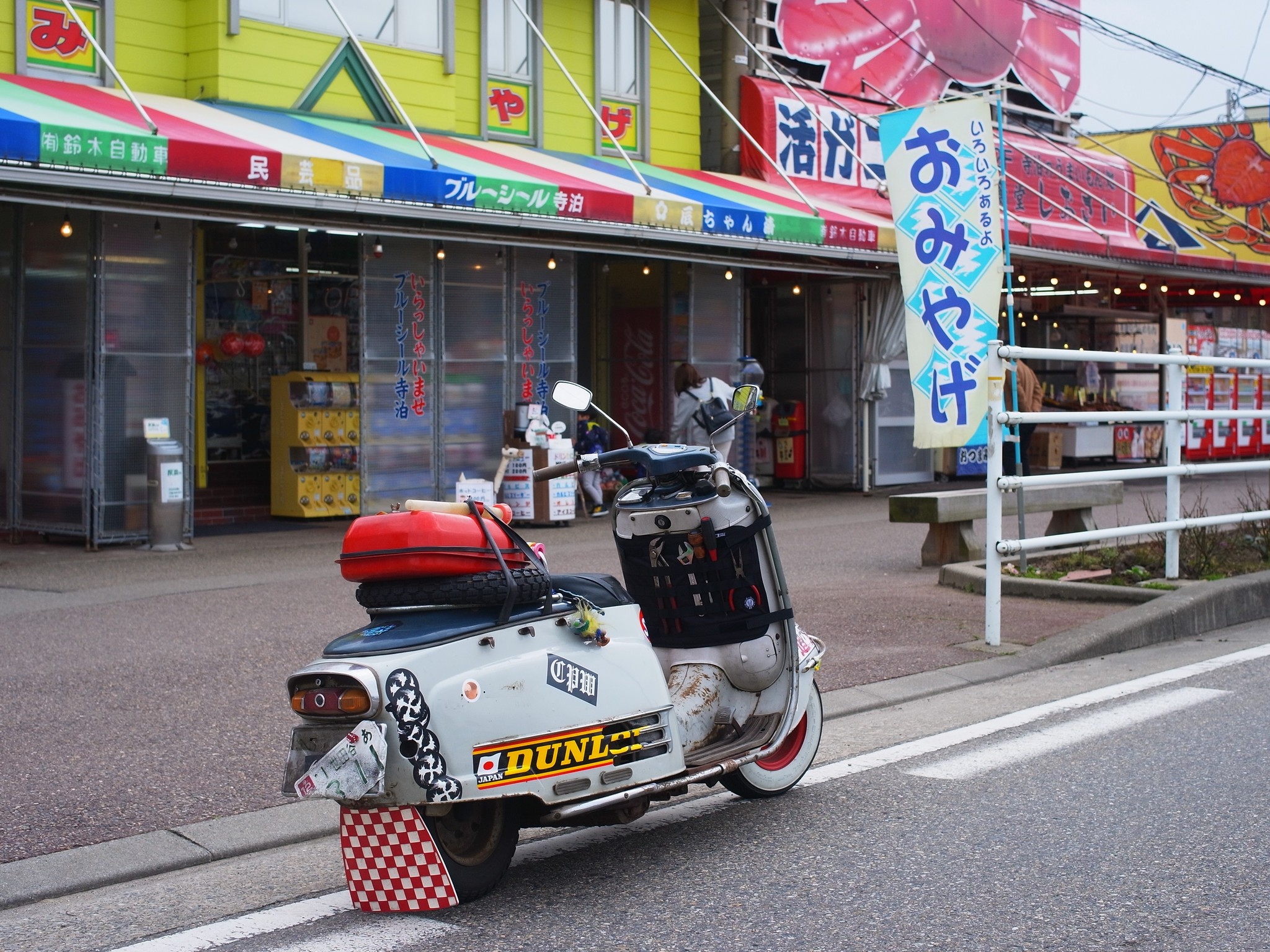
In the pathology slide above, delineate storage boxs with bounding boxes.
[337,509,522,583]
[1026,432,1064,469]
[501,411,577,525]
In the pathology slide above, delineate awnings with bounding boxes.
[0,66,902,265]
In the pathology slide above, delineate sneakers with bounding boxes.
[592,504,609,516]
[588,506,596,514]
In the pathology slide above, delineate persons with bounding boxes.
[572,411,611,517]
[670,363,758,462]
[1005,356,1043,477]
[635,428,665,478]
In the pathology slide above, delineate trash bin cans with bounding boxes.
[135,438,196,550]
[771,400,806,479]
[756,397,779,475]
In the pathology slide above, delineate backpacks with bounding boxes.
[681,375,735,436]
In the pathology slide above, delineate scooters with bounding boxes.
[279,378,827,913]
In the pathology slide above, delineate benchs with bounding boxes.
[888,480,1125,565]
